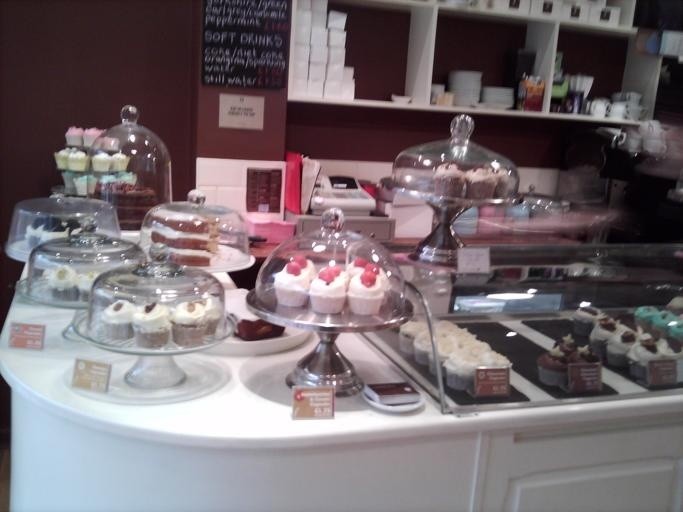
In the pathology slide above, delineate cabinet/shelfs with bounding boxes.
[289,0,643,123]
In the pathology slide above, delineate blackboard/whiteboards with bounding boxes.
[201,0,292,91]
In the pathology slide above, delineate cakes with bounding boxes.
[50,268,80,301]
[428,161,512,200]
[197,298,223,332]
[172,302,210,347]
[99,299,133,338]
[537,335,599,386]
[78,272,98,303]
[274,249,390,313]
[93,181,157,231]
[151,210,220,266]
[633,306,683,350]
[56,125,136,195]
[132,301,169,348]
[571,306,674,383]
[397,317,510,390]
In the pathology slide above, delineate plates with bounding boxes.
[482,84,515,110]
[448,70,482,107]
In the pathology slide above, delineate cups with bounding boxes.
[622,121,667,156]
[628,90,647,121]
[611,91,628,101]
[590,99,609,117]
[609,101,626,121]
